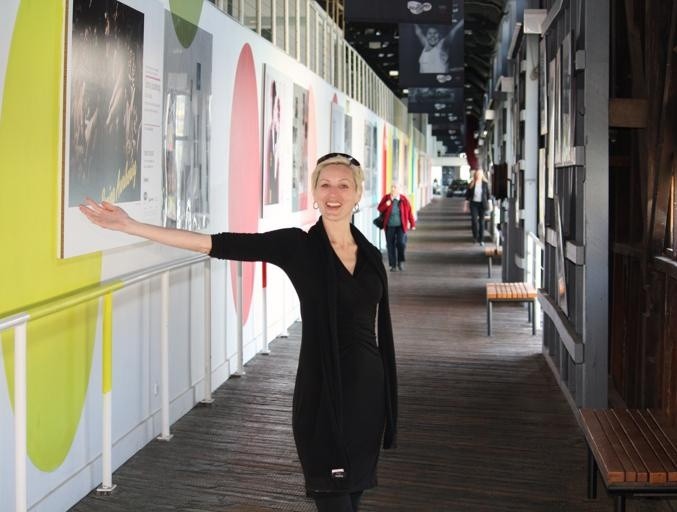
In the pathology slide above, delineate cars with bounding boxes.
[446,179,469,198]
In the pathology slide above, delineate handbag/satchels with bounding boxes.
[373,213,385,229]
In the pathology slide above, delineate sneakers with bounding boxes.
[473,238,484,246]
[390,262,405,271]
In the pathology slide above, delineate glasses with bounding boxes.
[317,153,360,166]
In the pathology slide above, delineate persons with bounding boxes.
[75,151,399,510]
[377,179,418,273]
[461,169,495,247]
[414,16,464,74]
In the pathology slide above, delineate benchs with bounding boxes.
[484,247,508,279]
[576,407,677,512]
[486,282,538,336]
